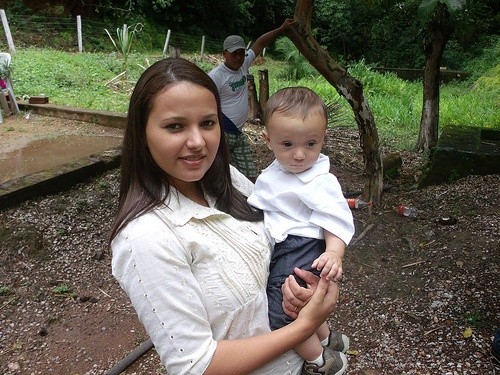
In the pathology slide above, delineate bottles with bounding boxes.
[393,206,418,218]
[346,199,372,209]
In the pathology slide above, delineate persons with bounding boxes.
[246,87,355,375]
[108,58,339,375]
[207,18,298,184]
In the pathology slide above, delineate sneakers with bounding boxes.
[301,346,347,375]
[325,328,349,353]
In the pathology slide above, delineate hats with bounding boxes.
[224,35,248,53]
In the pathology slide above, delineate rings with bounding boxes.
[294,307,297,312]
